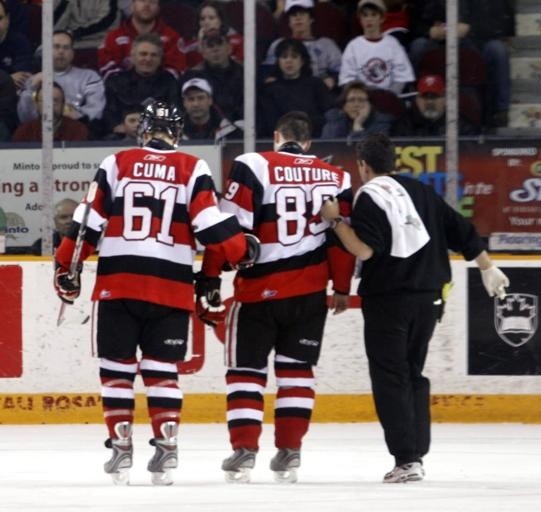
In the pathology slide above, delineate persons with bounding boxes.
[188,28,243,117]
[51,102,249,486]
[32,1,124,70]
[318,132,512,481]
[99,32,184,141]
[250,36,336,140]
[13,29,108,124]
[1,4,40,91]
[390,75,479,135]
[175,78,237,140]
[175,1,243,74]
[407,1,517,121]
[321,78,393,140]
[98,2,188,81]
[30,197,80,256]
[11,81,98,143]
[336,0,416,103]
[259,0,343,93]
[192,110,357,485]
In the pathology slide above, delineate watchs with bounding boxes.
[330,217,343,228]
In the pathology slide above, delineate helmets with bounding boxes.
[137,99,186,148]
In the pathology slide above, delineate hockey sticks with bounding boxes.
[56,180,98,328]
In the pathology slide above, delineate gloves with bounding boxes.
[193,272,226,328]
[52,262,81,305]
[231,233,261,270]
[480,265,510,300]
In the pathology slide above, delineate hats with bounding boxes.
[417,74,444,95]
[356,0,386,15]
[181,77,213,97]
[284,0,314,13]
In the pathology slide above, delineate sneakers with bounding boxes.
[269,448,301,473]
[221,446,257,472]
[382,461,423,482]
[103,439,132,474]
[146,439,178,473]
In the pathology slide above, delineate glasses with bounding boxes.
[345,96,369,104]
